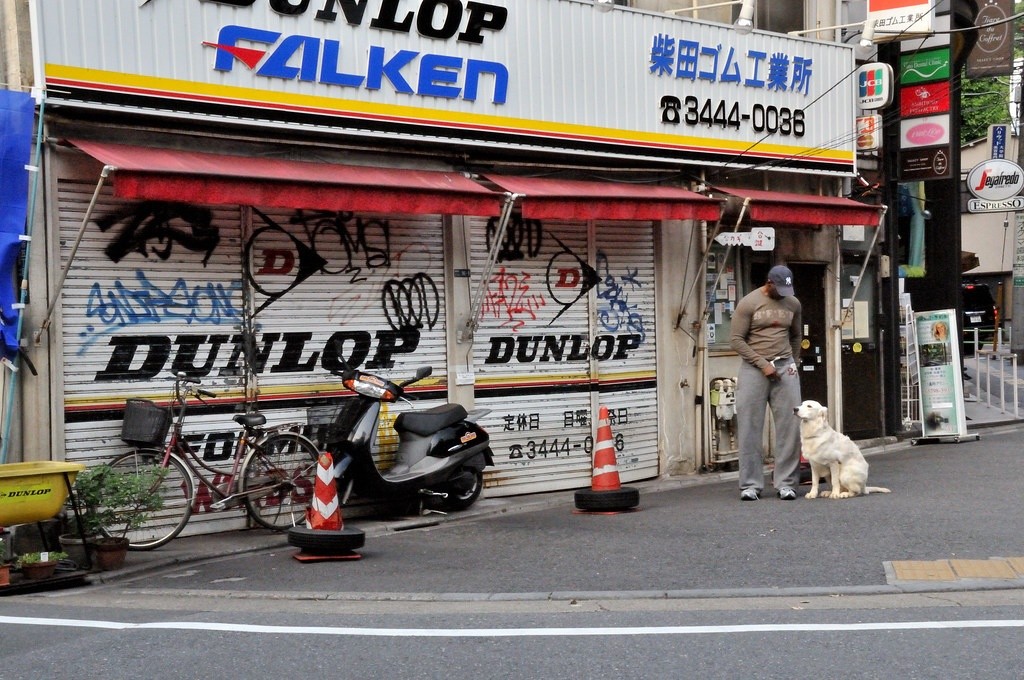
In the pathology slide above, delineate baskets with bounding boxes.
[121,398,174,447]
[306,401,358,445]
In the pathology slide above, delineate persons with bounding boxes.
[933,322,946,339]
[730,265,802,499]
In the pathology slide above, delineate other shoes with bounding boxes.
[777,486,796,500]
[741,488,761,501]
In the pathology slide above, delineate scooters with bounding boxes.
[307,340,495,517]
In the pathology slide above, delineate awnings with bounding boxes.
[37,139,512,345]
[700,185,888,327]
[472,174,729,330]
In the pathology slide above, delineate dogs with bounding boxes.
[793,399,892,499]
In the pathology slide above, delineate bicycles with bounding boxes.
[90,370,320,553]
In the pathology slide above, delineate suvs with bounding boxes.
[962,282,998,355]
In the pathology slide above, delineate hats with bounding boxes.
[768,266,794,296]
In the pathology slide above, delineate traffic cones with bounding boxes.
[285,454,367,563]
[573,406,641,514]
